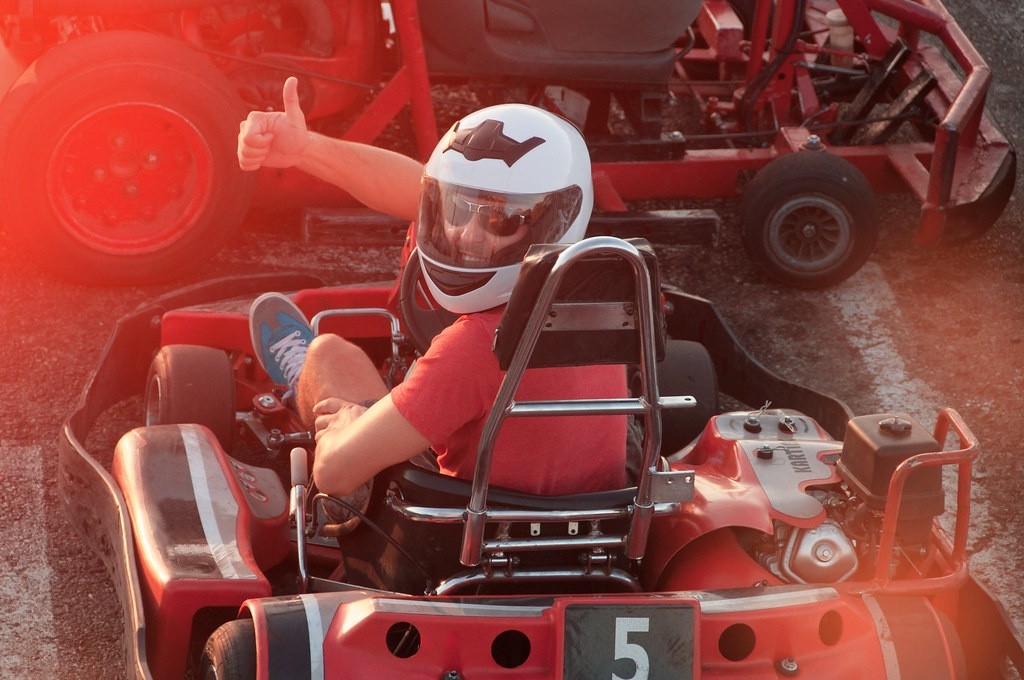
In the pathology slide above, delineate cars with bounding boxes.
[55,205,1024,680]
[0,0,1017,289]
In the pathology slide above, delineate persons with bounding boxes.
[237,76,627,493]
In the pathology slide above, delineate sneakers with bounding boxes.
[249,292,315,409]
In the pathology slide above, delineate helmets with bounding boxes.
[416,104,594,314]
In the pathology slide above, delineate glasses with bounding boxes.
[441,193,531,237]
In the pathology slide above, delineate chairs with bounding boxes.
[383,236,697,595]
[420,0,705,141]
